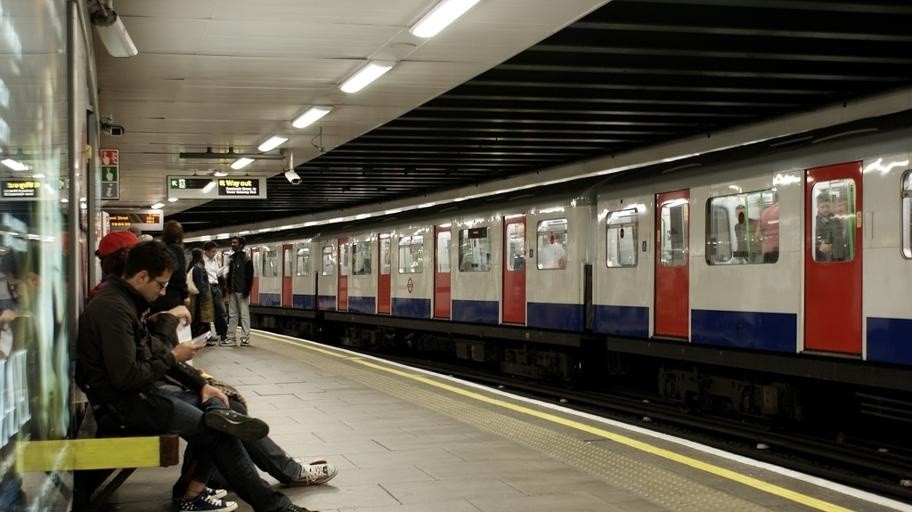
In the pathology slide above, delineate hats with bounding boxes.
[97,229,140,259]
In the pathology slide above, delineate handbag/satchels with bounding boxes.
[186,265,202,295]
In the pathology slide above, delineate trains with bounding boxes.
[182,122,911,460]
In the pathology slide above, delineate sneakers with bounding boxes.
[177,495,240,512]
[172,483,228,501]
[205,331,250,347]
[205,408,270,441]
[281,458,339,488]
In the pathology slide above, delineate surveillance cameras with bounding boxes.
[102,122,126,138]
[285,171,303,186]
[91,9,138,57]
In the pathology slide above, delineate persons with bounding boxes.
[163,222,254,346]
[1,247,41,512]
[75,230,338,511]
[734,190,848,263]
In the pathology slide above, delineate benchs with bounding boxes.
[13,398,181,512]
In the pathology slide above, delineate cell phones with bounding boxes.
[190,330,213,344]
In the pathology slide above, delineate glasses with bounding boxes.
[150,274,169,289]
